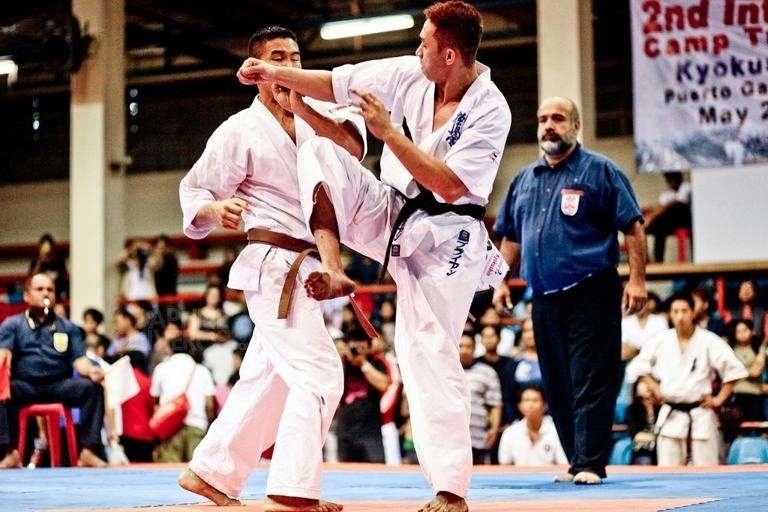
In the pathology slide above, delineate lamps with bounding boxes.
[320,12,414,42]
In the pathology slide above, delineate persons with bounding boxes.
[1,229,767,468]
[179,27,367,512]
[237,0,512,512]
[644,170,692,263]
[493,97,649,485]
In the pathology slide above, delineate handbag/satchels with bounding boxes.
[149,393,190,440]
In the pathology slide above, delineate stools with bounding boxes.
[634,207,689,263]
[18,404,78,467]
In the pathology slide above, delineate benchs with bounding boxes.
[0,241,69,320]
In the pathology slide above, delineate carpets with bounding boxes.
[41,497,723,512]
